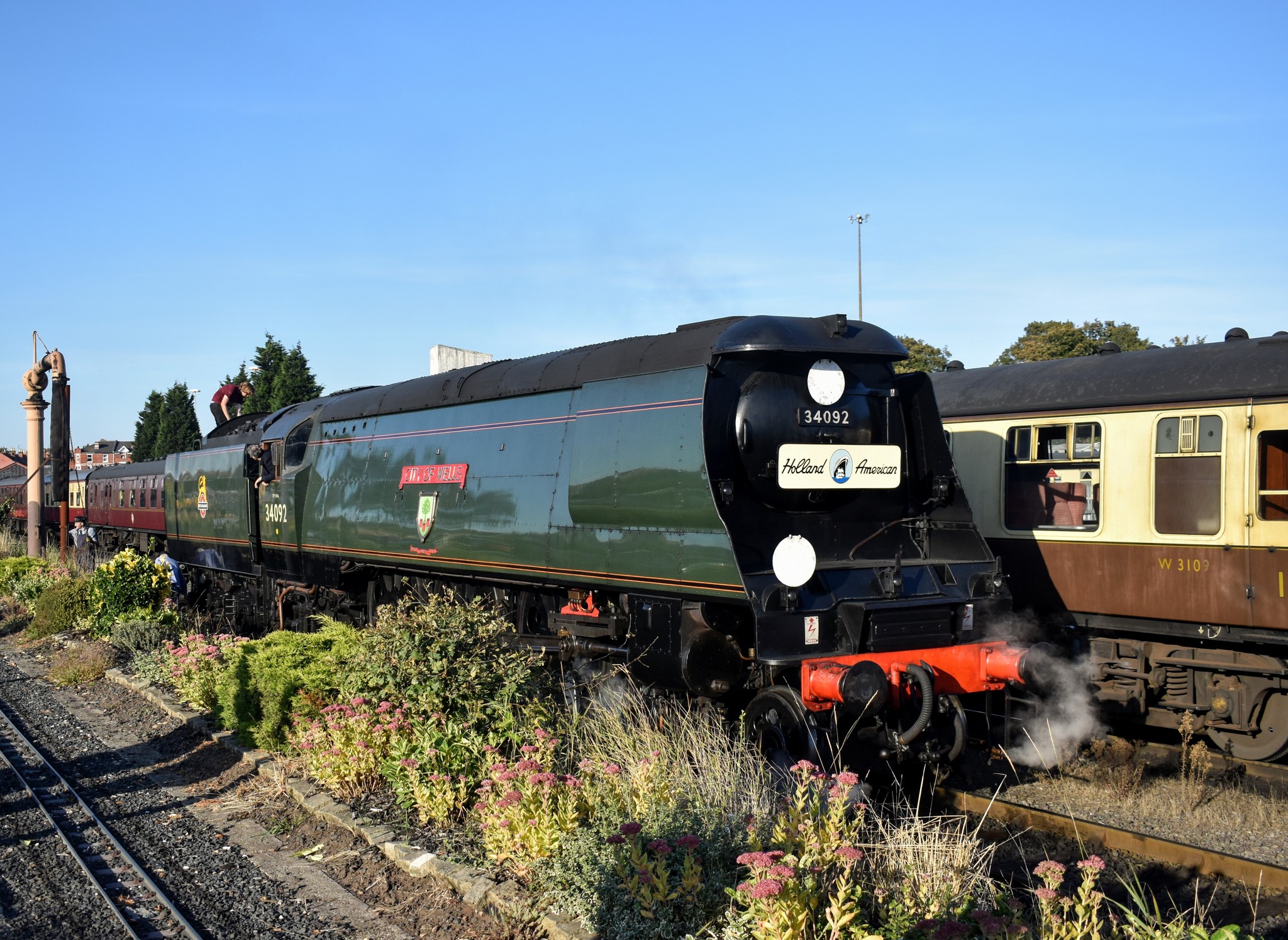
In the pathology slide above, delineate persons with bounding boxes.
[153,551,186,606]
[191,546,225,572]
[209,381,255,427]
[244,442,278,489]
[66,516,98,574]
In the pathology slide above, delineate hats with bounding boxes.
[193,545,203,552]
[159,551,169,557]
[72,516,87,523]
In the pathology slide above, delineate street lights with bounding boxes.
[847,212,871,322]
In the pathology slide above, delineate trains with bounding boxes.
[920,327,1288,764]
[0,313,1061,800]
